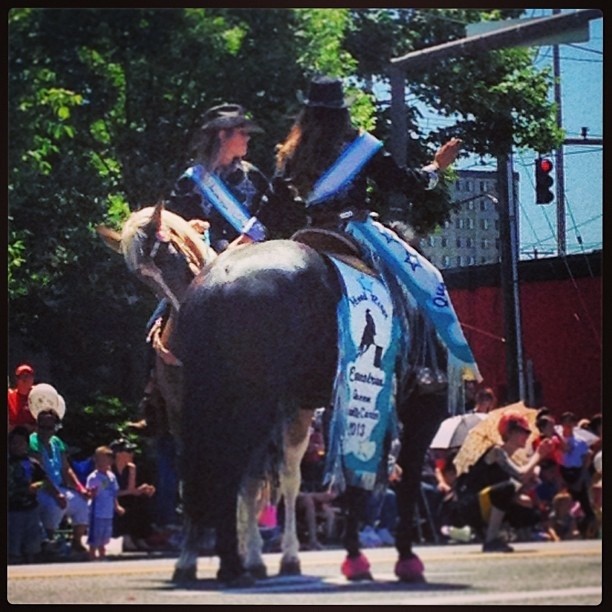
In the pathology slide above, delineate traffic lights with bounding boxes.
[535,158,554,204]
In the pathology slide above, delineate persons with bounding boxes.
[145,107,272,342]
[359,464,402,545]
[532,407,568,479]
[434,460,451,491]
[85,446,125,560]
[228,78,483,381]
[295,432,341,547]
[110,439,155,553]
[7,429,48,563]
[6,366,65,431]
[444,419,553,552]
[563,409,602,537]
[467,388,498,414]
[546,494,574,541]
[31,411,92,560]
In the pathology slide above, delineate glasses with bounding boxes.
[518,425,531,436]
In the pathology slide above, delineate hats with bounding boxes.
[109,438,137,450]
[296,77,354,109]
[202,104,265,134]
[15,365,33,376]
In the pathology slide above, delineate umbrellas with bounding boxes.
[453,401,543,477]
[430,412,487,449]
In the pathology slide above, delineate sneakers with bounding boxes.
[483,537,513,551]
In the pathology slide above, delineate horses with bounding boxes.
[95,197,317,589]
[171,221,447,582]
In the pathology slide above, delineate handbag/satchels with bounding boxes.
[442,444,494,516]
